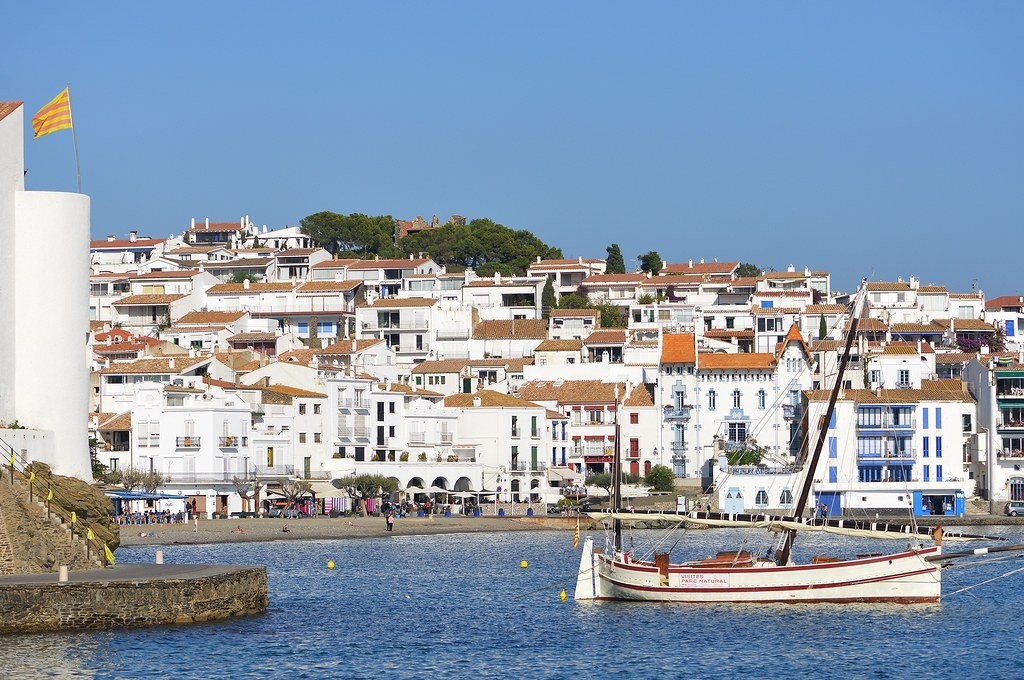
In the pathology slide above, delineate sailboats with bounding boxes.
[596,280,944,605]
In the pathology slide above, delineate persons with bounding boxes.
[382,498,475,518]
[192,498,196,515]
[820,502,828,518]
[113,504,183,524]
[564,484,586,495]
[283,523,290,533]
[186,502,191,520]
[231,525,246,534]
[706,501,712,517]
[561,500,574,517]
[386,513,394,532]
[193,517,199,533]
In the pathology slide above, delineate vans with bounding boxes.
[1004,502,1024,516]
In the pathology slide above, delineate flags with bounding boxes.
[573,517,578,549]
[32,87,72,140]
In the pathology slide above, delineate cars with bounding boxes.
[268,504,303,519]
[547,503,559,513]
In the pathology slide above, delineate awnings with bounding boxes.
[549,468,584,482]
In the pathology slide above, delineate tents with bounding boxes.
[101,491,189,525]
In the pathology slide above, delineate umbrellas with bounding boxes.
[402,484,477,515]
